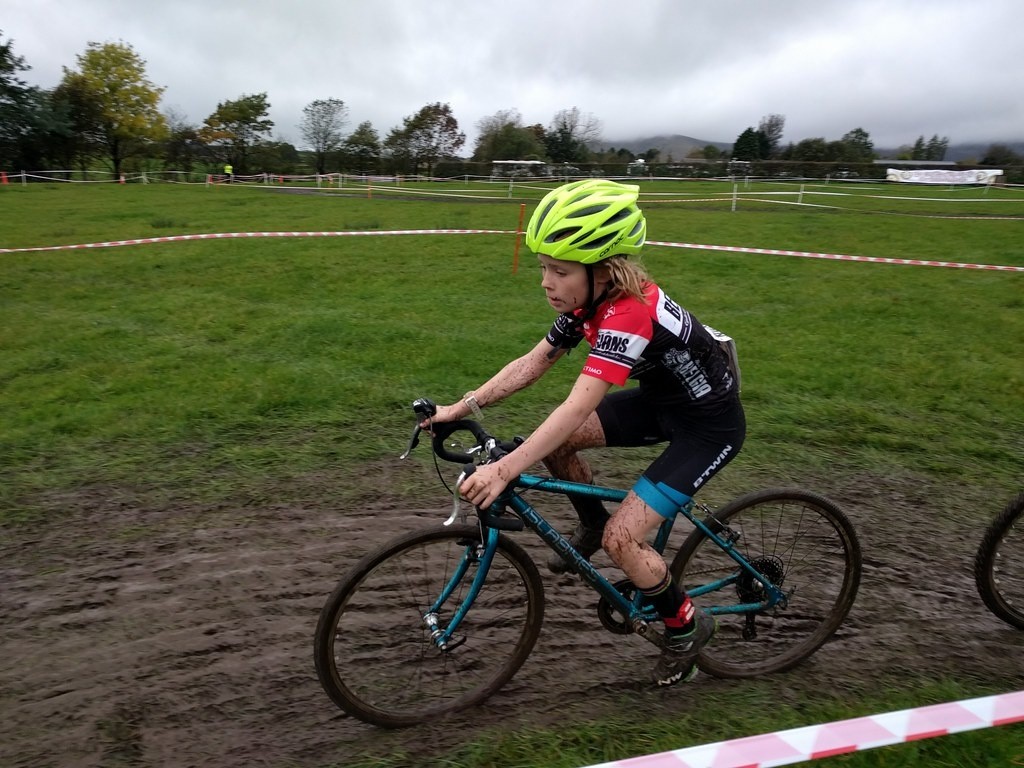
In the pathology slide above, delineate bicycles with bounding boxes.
[312,394,863,731]
[974,494,1024,633]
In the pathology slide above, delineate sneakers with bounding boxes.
[649,611,719,689]
[549,517,619,574]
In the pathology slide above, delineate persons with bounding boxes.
[412,179,748,687]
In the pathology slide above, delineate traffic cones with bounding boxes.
[1,172,10,185]
[119,172,126,185]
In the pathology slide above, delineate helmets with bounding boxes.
[524,179,646,263]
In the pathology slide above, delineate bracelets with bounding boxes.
[462,391,484,421]
